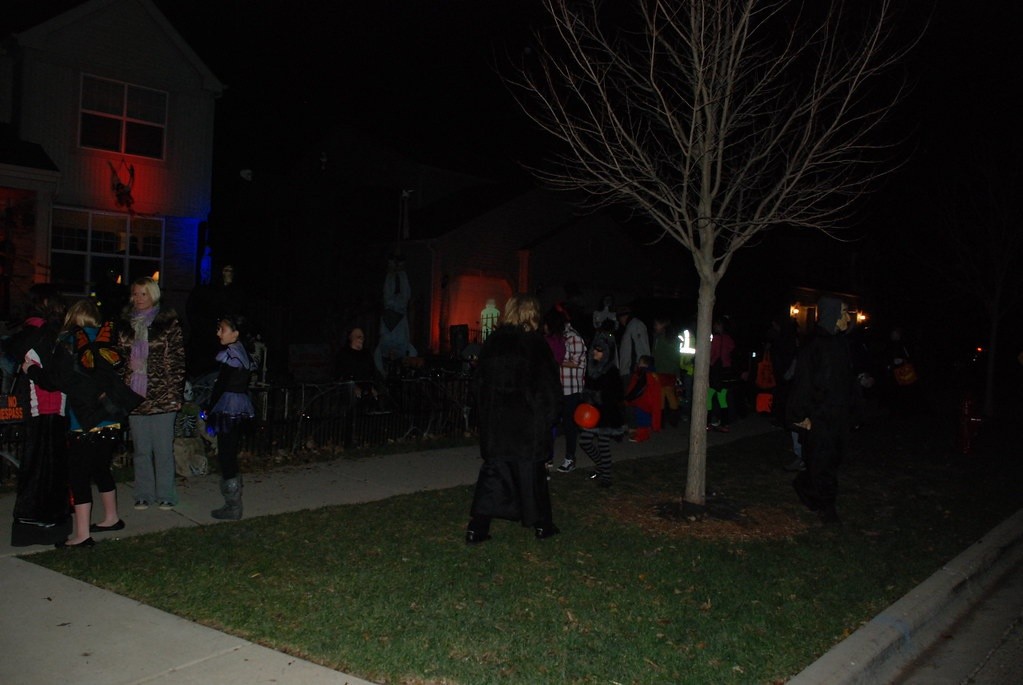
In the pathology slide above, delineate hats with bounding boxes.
[615,305,633,315]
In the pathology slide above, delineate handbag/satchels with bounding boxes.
[756,345,775,387]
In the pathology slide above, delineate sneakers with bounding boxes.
[90,518,125,531]
[54,537,96,549]
[465,532,493,543]
[781,453,839,524]
[157,501,175,509]
[536,527,561,538]
[134,501,149,510]
[545,414,731,490]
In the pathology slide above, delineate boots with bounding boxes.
[211,477,243,519]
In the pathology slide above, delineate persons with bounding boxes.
[743,319,926,478]
[1,276,253,549]
[462,291,738,546]
[333,326,379,450]
[790,296,871,522]
